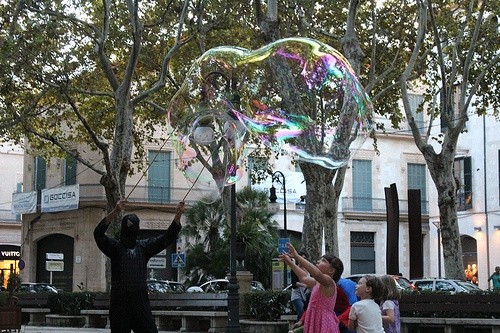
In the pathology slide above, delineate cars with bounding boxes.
[11,282,63,309]
[408,279,484,294]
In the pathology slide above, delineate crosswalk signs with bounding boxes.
[172,254,186,268]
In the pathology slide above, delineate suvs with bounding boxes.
[343,273,419,298]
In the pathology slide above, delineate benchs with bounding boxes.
[400,294,500,333]
[16,294,52,325]
[79,294,231,333]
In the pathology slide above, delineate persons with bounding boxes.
[488,266,500,292]
[291,252,313,322]
[93,199,185,333]
[279,242,343,333]
[294,275,402,333]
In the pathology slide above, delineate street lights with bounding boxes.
[269,170,290,288]
[432,221,441,279]
[186,61,248,333]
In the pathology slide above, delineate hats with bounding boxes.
[495,267,500,271]
